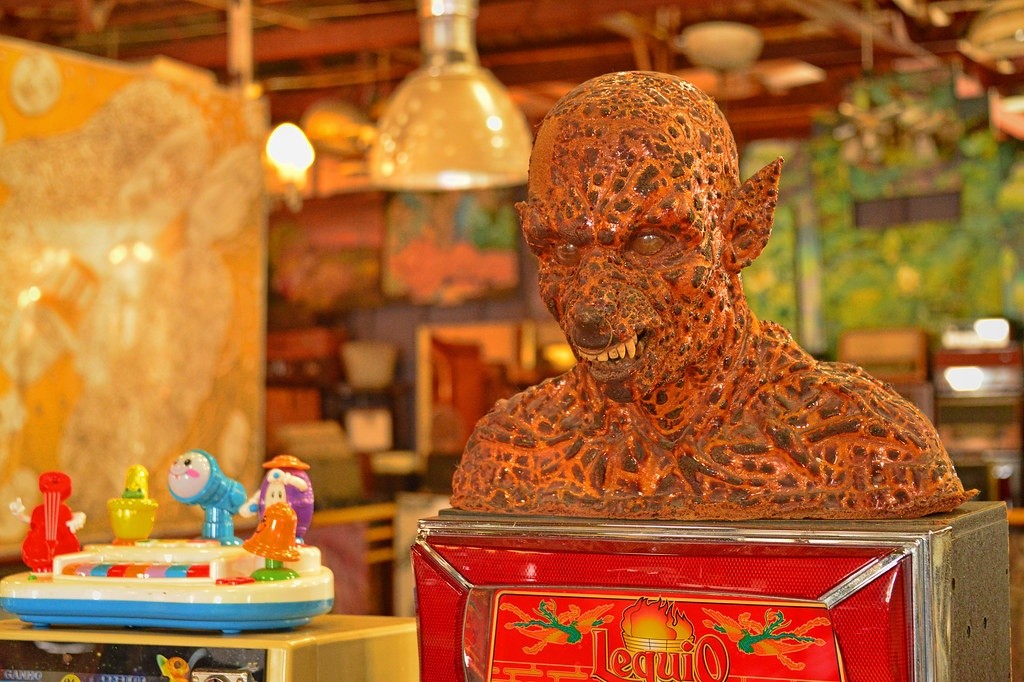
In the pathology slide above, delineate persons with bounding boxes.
[448,70,978,517]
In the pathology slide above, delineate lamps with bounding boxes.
[367,0,533,195]
